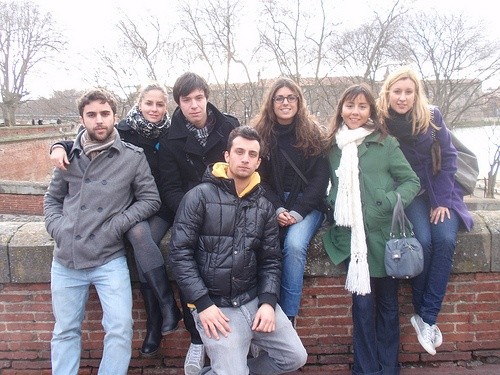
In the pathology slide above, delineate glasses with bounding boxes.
[272,95,299,103]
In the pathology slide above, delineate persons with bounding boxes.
[154,71,241,375]
[48,83,185,357]
[31,117,63,126]
[43,87,163,375]
[251,78,328,328]
[372,66,474,357]
[164,121,312,374]
[318,85,422,374]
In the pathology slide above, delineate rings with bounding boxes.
[59,161,63,165]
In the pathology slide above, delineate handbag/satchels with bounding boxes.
[430,106,479,196]
[385,192,424,279]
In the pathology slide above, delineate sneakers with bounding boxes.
[410,314,443,355]
[183,341,206,375]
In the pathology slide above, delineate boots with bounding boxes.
[140,282,162,356]
[145,266,183,335]
